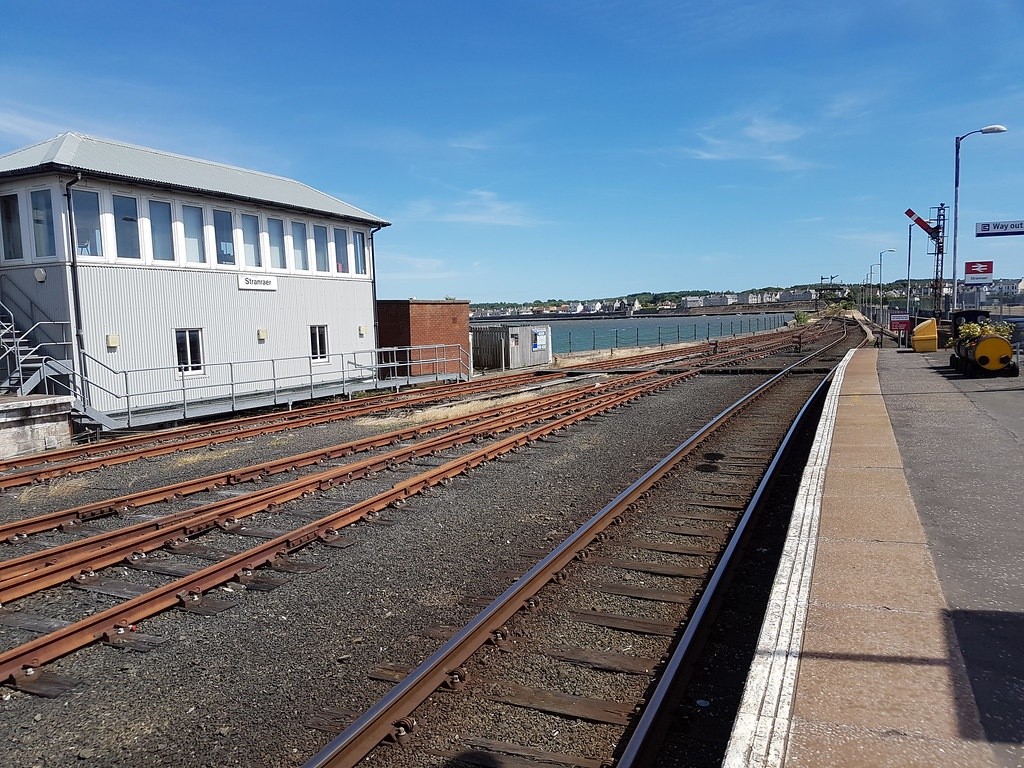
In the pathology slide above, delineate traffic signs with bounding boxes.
[965,260,993,284]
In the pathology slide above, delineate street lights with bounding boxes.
[907,219,934,315]
[858,264,880,323]
[880,249,896,326]
[952,125,1007,313]
